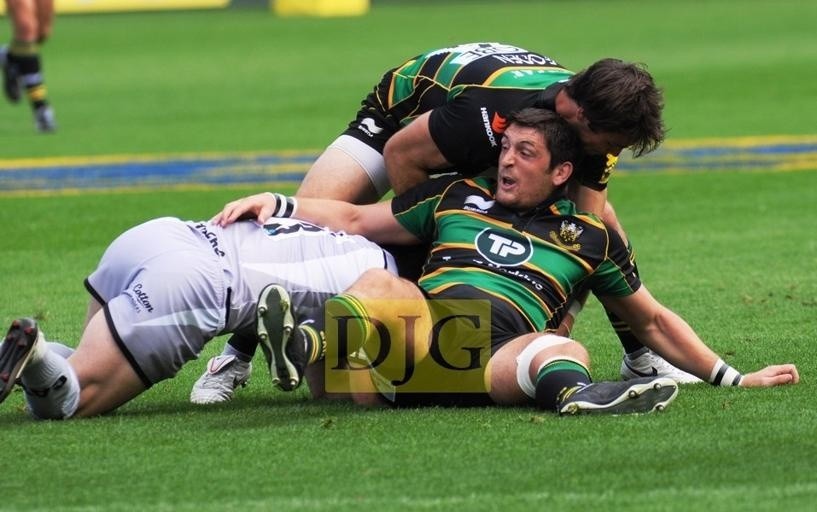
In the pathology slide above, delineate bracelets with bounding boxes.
[558,320,570,337]
[705,359,743,388]
[267,191,296,217]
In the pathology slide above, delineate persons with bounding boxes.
[207,107,800,418]
[0,213,413,421]
[187,41,702,405]
[0,0,61,133]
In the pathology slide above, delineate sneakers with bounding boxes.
[557,375,678,415]
[190,354,253,405]
[254,283,307,391]
[621,350,703,385]
[0,317,40,402]
